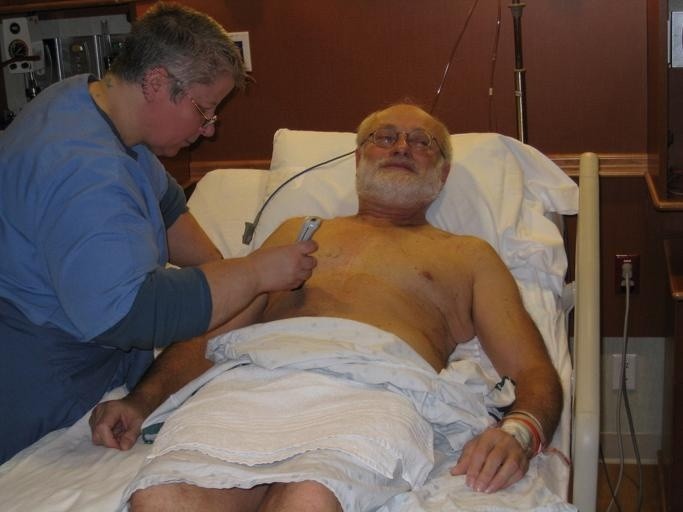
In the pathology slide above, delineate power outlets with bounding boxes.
[610,352,638,394]
[613,254,637,295]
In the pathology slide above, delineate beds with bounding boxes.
[0,152,603,511]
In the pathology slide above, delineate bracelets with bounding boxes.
[500,412,548,459]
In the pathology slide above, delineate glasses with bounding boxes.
[162,66,217,129]
[357,128,447,159]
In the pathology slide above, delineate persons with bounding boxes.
[88,101,563,512]
[0,2,317,467]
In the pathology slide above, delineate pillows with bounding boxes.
[246,126,578,290]
